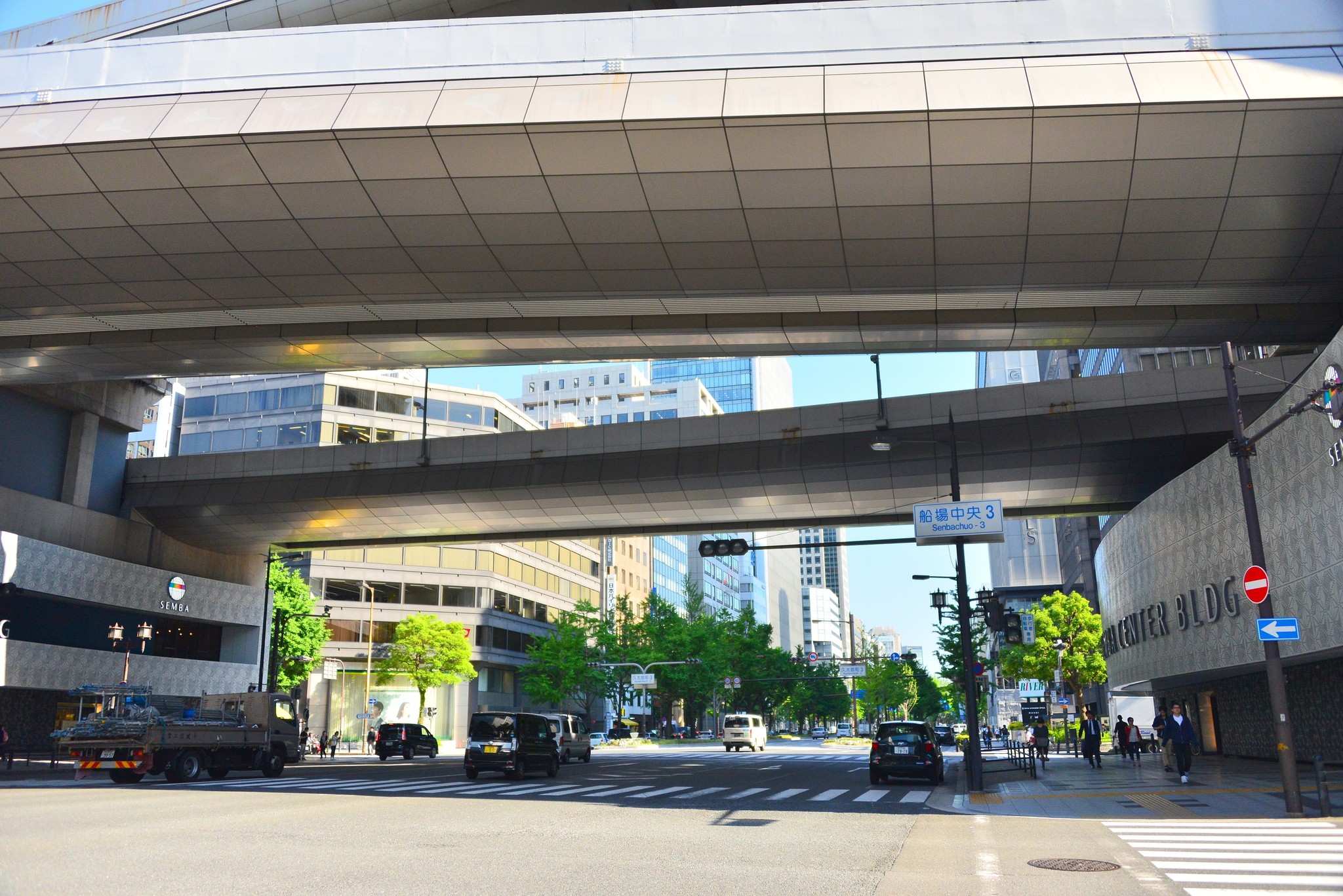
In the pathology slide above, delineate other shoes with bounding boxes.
[1090,764,1094,768]
[367,753,373,756]
[1045,757,1050,761]
[1036,753,1040,759]
[298,751,337,760]
[1165,765,1174,772]
[1097,763,1102,768]
[1122,756,1126,758]
[1132,760,1140,766]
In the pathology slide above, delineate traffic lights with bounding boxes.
[901,653,917,659]
[684,659,701,664]
[585,661,601,667]
[698,538,749,558]
[788,658,803,662]
[427,706,437,733]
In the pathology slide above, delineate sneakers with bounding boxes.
[1181,776,1188,783]
[1184,771,1189,778]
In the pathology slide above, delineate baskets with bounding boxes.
[1037,736,1048,746]
[1048,736,1056,743]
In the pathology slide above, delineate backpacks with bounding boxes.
[982,728,987,736]
[328,735,334,746]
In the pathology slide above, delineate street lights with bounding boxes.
[811,612,860,738]
[911,559,1002,765]
[107,621,154,685]
[869,402,987,795]
[1051,638,1072,755]
[355,581,375,755]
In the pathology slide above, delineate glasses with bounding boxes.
[1172,707,1180,709]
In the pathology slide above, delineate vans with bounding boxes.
[464,711,560,781]
[374,723,439,759]
[537,713,591,763]
[722,714,769,751]
[836,723,853,738]
[953,724,967,733]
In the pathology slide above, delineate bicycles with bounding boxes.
[1036,736,1048,769]
[1169,740,1202,756]
[1026,736,1056,754]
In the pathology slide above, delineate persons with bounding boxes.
[1114,715,1131,758]
[367,727,376,756]
[306,732,316,754]
[0,724,8,762]
[298,727,309,761]
[367,701,386,731]
[330,731,341,760]
[1152,705,1175,773]
[1162,702,1198,783]
[979,710,1108,769]
[319,731,327,759]
[1125,717,1143,767]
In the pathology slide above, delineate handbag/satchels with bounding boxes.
[1114,737,1119,747]
[1030,735,1035,743]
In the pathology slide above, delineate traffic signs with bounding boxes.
[369,699,376,703]
[890,653,900,662]
[1256,617,1300,641]
[1058,698,1069,704]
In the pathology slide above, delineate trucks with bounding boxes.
[60,683,310,779]
[1106,695,1161,754]
[858,723,874,737]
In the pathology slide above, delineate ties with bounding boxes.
[1088,720,1092,738]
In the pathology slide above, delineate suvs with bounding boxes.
[671,731,723,740]
[608,728,632,739]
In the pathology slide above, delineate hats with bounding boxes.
[309,732,312,735]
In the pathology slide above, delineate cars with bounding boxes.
[588,732,612,749]
[638,733,660,741]
[869,720,944,785]
[767,728,814,736]
[934,725,955,745]
[811,727,829,739]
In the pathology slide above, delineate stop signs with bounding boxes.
[973,662,983,675]
[1242,565,1271,604]
